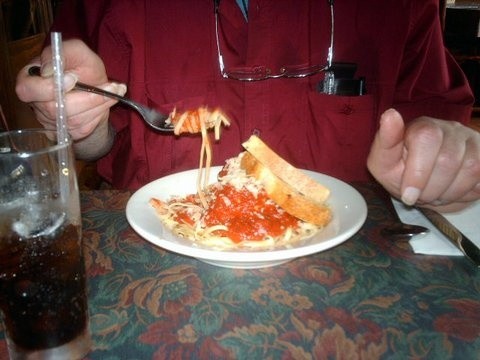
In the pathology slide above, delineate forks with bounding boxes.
[25,65,176,133]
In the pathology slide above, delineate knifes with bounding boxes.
[416,207,480,268]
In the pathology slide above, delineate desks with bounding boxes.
[0,181,480,360]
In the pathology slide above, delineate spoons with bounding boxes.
[370,177,430,241]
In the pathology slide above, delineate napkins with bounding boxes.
[390,196,480,257]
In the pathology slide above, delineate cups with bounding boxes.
[0,127,92,360]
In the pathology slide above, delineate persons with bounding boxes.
[15,0,480,213]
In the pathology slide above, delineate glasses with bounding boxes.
[215,4,334,81]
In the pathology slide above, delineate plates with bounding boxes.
[125,165,368,269]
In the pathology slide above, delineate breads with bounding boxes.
[239,135,333,227]
